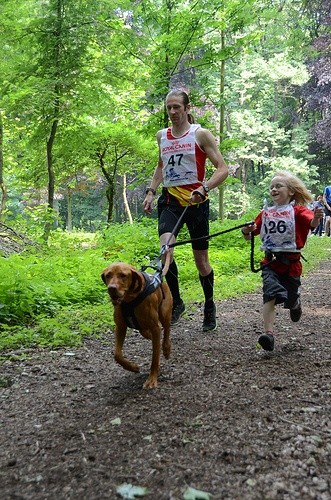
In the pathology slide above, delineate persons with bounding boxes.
[307,185,331,237]
[242,170,323,351]
[141,89,228,332]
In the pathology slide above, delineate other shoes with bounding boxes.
[291,306,301,322]
[171,303,186,325]
[258,331,276,351]
[202,306,217,332]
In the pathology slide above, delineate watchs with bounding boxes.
[203,185,209,192]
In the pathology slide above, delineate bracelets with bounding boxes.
[242,231,249,235]
[146,188,155,195]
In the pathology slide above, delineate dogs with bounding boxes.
[99,258,175,393]
